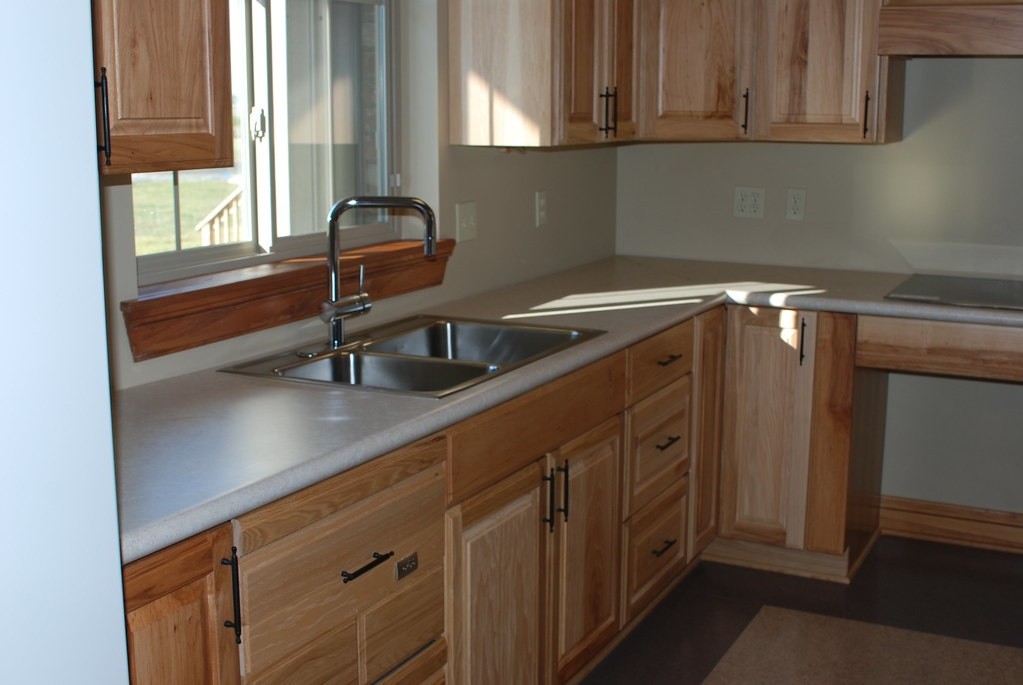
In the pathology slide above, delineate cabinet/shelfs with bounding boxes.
[124,304,860,685]
[90,0,234,176]
[446,0,904,148]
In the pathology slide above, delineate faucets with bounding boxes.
[324,194,438,351]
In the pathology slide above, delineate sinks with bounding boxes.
[273,348,499,396]
[359,319,586,367]
